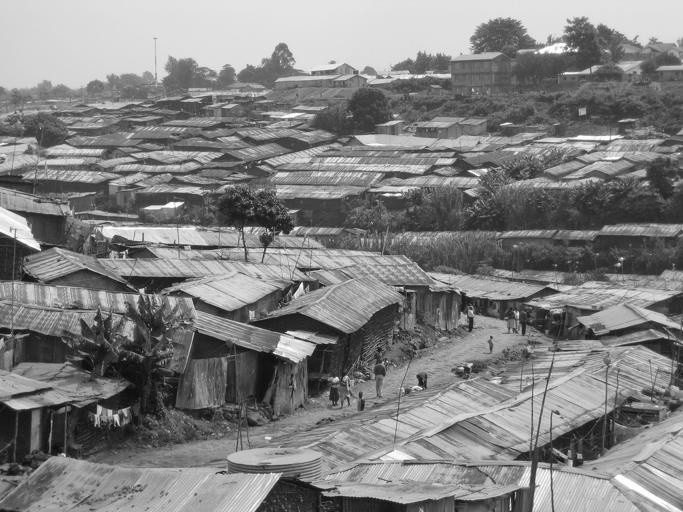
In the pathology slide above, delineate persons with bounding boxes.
[328,369,350,409]
[488,336,493,353]
[468,306,474,332]
[507,306,527,336]
[416,372,427,388]
[358,392,365,411]
[374,347,386,398]
